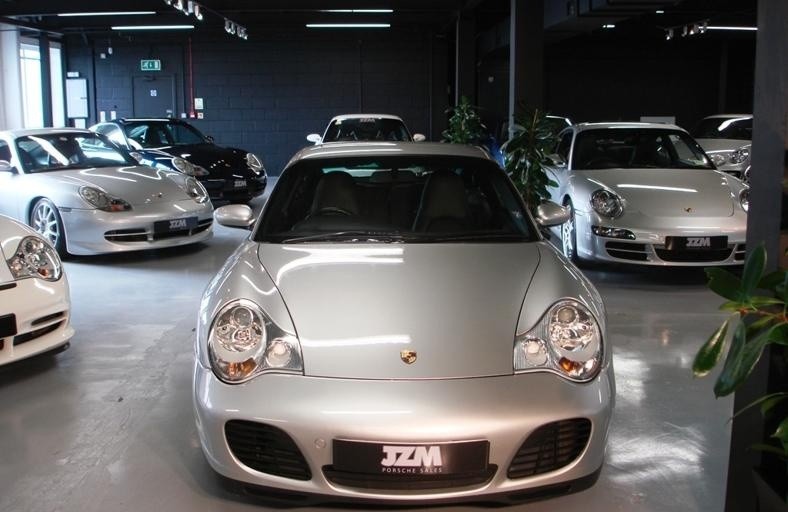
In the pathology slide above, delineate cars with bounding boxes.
[0,215,74,368]
[0,128,215,259]
[539,111,751,273]
[115,117,267,203]
[308,114,427,178]
[193,141,615,511]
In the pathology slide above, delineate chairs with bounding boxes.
[413,170,470,228]
[311,171,359,217]
[631,135,665,167]
